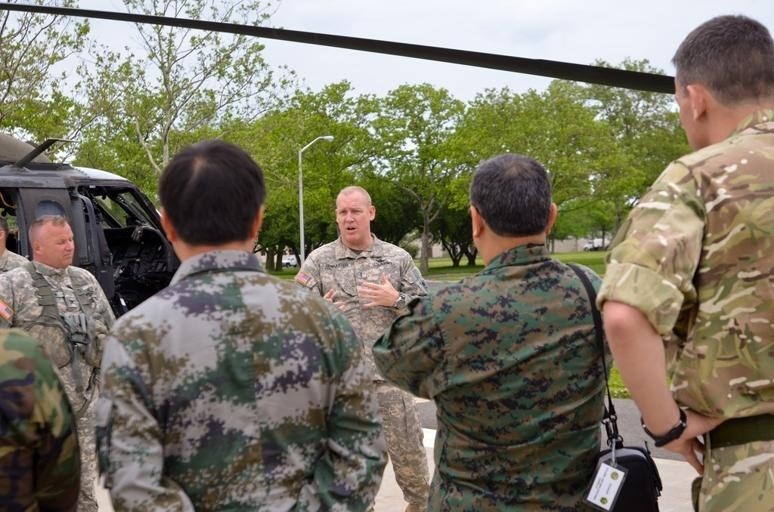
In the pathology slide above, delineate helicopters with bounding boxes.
[0,0,677,323]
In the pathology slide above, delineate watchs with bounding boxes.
[393,292,406,309]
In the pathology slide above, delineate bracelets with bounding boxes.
[640,407,687,447]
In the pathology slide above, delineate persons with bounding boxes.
[371,153,613,512]
[294,185,430,512]
[595,14,774,512]
[95,140,388,512]
[0,214,117,512]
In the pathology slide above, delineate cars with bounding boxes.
[281,255,301,268]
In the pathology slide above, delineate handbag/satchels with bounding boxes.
[584,446,662,512]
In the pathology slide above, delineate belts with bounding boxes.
[695,412,774,451]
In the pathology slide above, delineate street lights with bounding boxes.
[297,136,335,270]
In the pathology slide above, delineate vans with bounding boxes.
[583,239,609,252]
